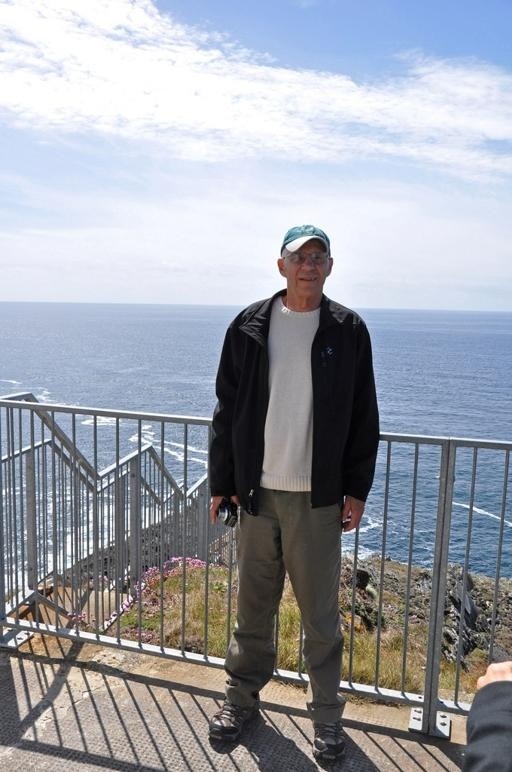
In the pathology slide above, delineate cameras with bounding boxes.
[215,499,238,527]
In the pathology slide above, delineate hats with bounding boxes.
[281,224,330,253]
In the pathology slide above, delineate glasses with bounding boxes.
[288,252,328,265]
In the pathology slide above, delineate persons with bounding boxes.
[458,661,512,771]
[206,224,382,763]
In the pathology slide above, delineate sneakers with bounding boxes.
[209,700,260,740]
[312,720,345,759]
